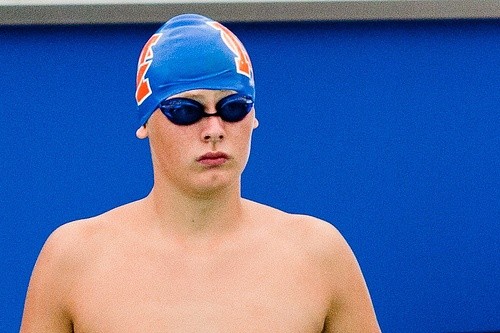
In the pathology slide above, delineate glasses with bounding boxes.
[158,92,254,126]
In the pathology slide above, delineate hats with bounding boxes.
[135,13,257,131]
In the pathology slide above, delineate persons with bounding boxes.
[19,12,382,333]
